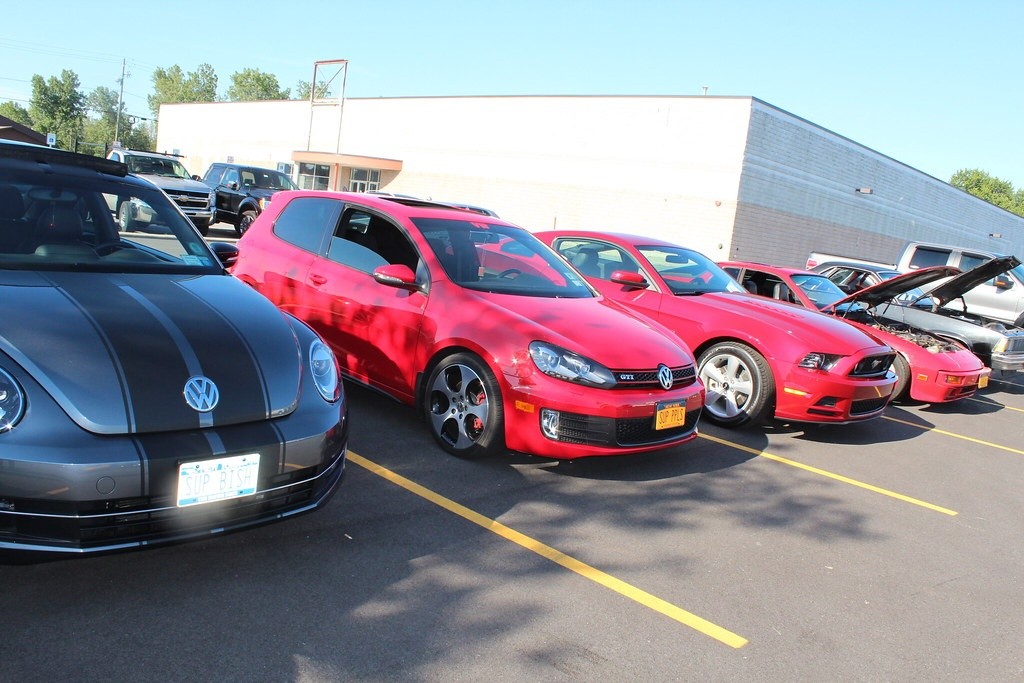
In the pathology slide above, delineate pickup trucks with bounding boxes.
[99,150,219,238]
[200,163,302,240]
[804,241,1024,329]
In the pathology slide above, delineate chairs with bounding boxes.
[572,248,601,278]
[0,186,84,254]
[743,281,757,294]
[773,283,788,301]
[137,159,163,174]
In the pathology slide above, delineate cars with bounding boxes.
[793,253,1024,371]
[0,138,350,566]
[657,259,992,404]
[471,231,898,433]
[350,202,505,240]
[228,190,707,463]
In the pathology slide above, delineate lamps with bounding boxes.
[989,233,1002,238]
[856,188,873,194]
